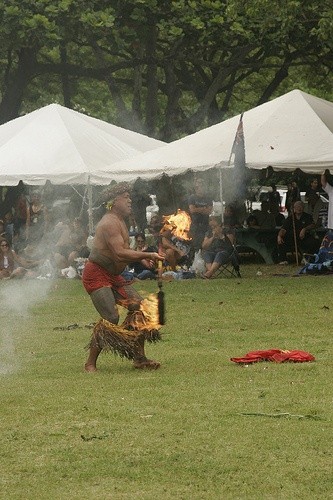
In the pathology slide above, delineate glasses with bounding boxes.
[136,240,146,243]
[1,244,8,247]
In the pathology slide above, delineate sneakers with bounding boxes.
[36,275,47,280]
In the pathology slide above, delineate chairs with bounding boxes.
[213,229,239,279]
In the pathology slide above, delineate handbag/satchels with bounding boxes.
[231,247,241,272]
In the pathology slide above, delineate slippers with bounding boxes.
[202,273,215,280]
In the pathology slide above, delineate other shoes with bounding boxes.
[280,261,288,265]
[134,355,160,369]
[85,362,98,373]
[300,259,308,265]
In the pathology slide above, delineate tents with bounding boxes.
[0,103,169,186]
[88,89,333,186]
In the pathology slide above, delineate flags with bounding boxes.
[231,121,245,169]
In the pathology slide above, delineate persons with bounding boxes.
[82,181,165,372]
[0,172,333,279]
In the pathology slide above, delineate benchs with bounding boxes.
[235,244,316,264]
